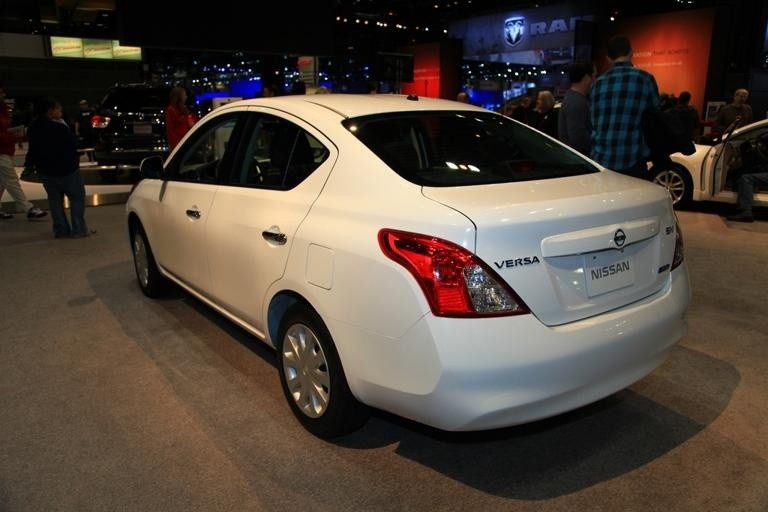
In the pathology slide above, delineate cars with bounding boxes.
[647,119,766,216]
[123,90,691,437]
[459,76,507,111]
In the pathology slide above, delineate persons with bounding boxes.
[662,88,766,220]
[587,35,664,181]
[513,62,613,150]
[454,92,469,102]
[0,95,97,220]
[27,97,89,239]
[258,124,295,186]
[262,85,275,96]
[165,84,199,173]
[315,84,330,94]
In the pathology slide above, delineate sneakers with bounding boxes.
[0,207,48,221]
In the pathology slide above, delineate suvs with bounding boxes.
[89,76,216,174]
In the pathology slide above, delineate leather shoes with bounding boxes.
[726,214,754,222]
